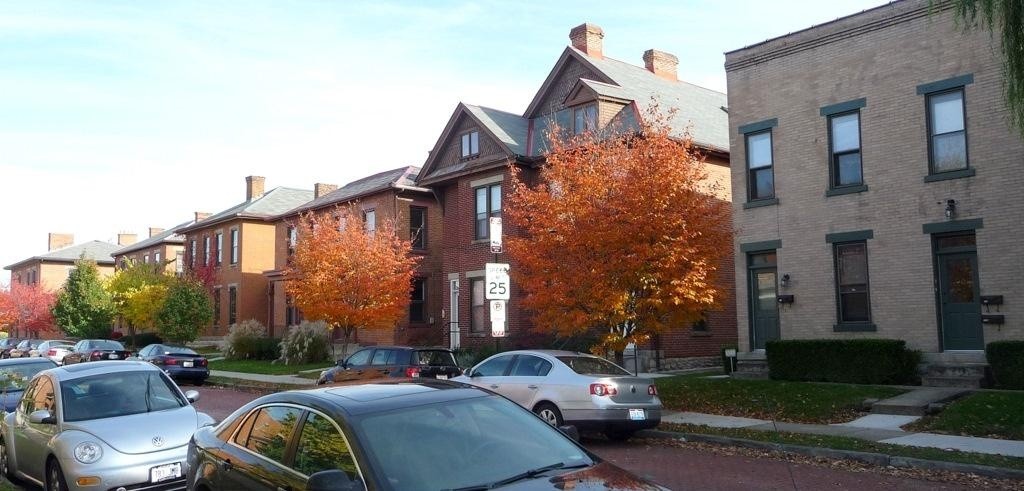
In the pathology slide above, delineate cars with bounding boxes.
[2,333,217,490]
[185,380,674,491]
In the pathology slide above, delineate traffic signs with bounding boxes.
[486,263,510,301]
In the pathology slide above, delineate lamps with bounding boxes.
[945,200,955,219]
[781,273,790,289]
